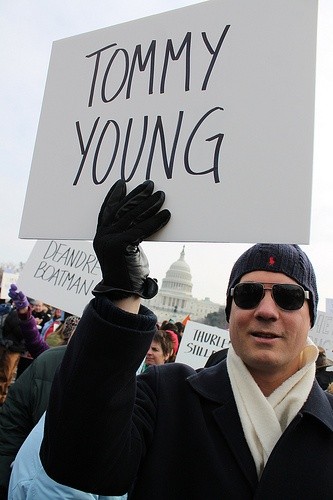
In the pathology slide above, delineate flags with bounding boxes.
[182,316,191,327]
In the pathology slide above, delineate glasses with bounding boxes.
[230,283,312,312]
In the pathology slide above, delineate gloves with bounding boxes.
[8,285,30,311]
[92,179,171,300]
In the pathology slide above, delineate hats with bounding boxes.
[166,330,178,356]
[175,322,185,333]
[318,371,333,390]
[225,243,319,330]
[168,318,175,324]
[63,316,80,339]
[315,347,333,368]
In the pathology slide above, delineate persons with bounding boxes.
[0,284,82,406]
[0,345,68,500]
[8,409,128,500]
[136,319,186,376]
[39,179,333,500]
